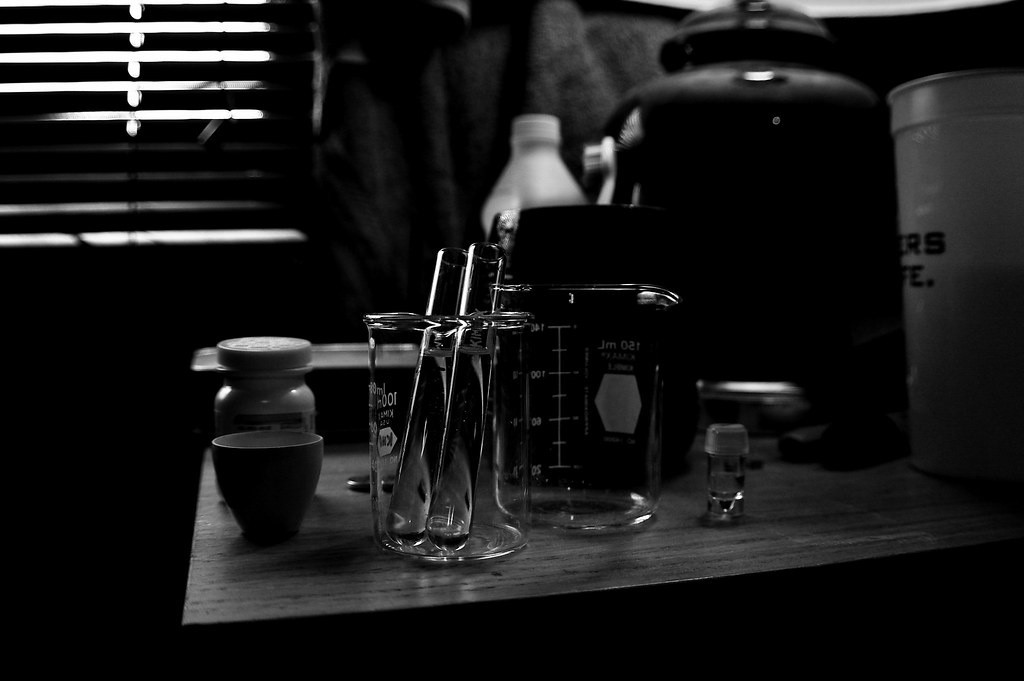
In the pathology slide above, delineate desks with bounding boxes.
[179,439,1024,628]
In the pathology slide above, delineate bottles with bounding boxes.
[213,336,315,436]
[481,114,590,251]
[704,423,749,516]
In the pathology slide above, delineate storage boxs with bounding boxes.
[190,341,421,443]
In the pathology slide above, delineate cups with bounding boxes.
[212,431,322,538]
[363,313,536,560]
[888,71,1023,480]
[490,284,683,531]
[515,207,696,479]
